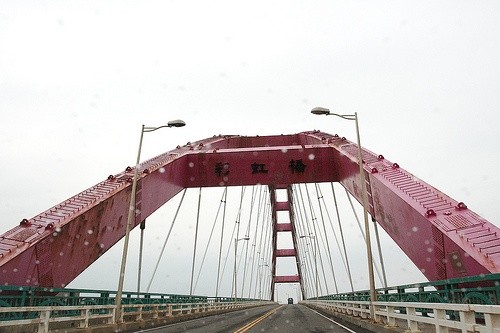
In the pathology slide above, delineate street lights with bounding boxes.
[312,106,380,327]
[299,234,319,300]
[231,235,249,297]
[112,120,187,324]
